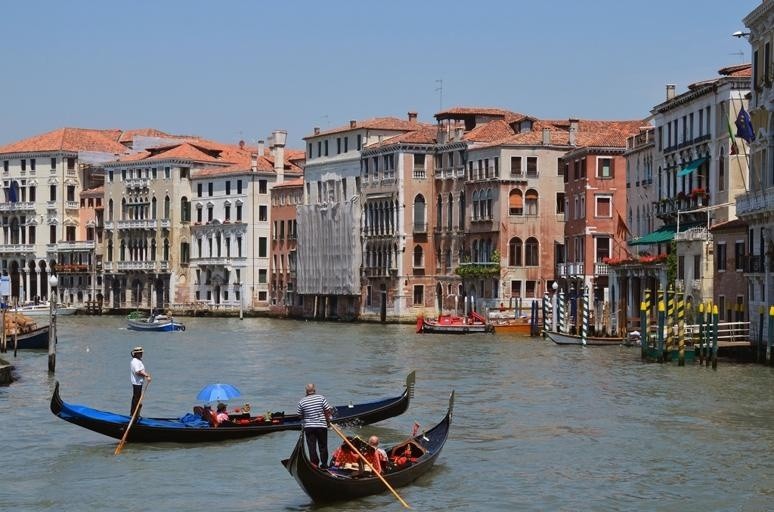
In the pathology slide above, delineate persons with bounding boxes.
[96,290,104,313]
[297,382,332,472]
[130,346,151,421]
[500,302,506,311]
[193,403,231,427]
[331,434,389,475]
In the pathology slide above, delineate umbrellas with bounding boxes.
[196,383,241,402]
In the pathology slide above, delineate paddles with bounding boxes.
[114,381,151,453]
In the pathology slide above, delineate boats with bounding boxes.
[279,387,457,501]
[491,321,543,337]
[420,310,489,335]
[0,295,78,350]
[51,368,416,445]
[127,317,185,332]
[545,328,632,347]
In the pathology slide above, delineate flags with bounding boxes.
[724,103,756,156]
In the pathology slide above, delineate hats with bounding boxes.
[133,347,144,353]
[217,403,228,409]
